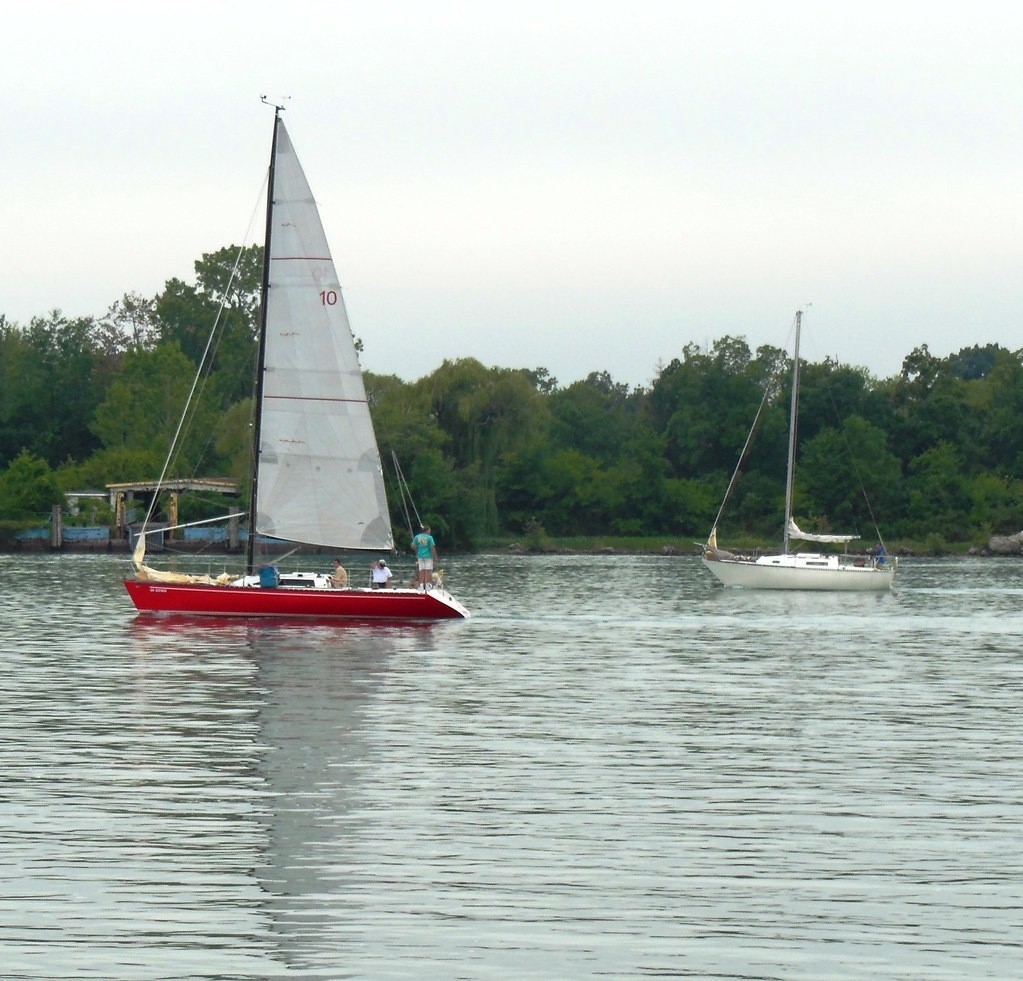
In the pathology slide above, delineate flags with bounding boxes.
[707,529,717,550]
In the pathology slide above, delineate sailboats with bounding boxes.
[132,615,460,973]
[121,92,473,620]
[702,311,899,591]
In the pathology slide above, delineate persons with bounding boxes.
[411,525,437,590]
[874,543,884,571]
[373,559,393,589]
[329,559,347,589]
